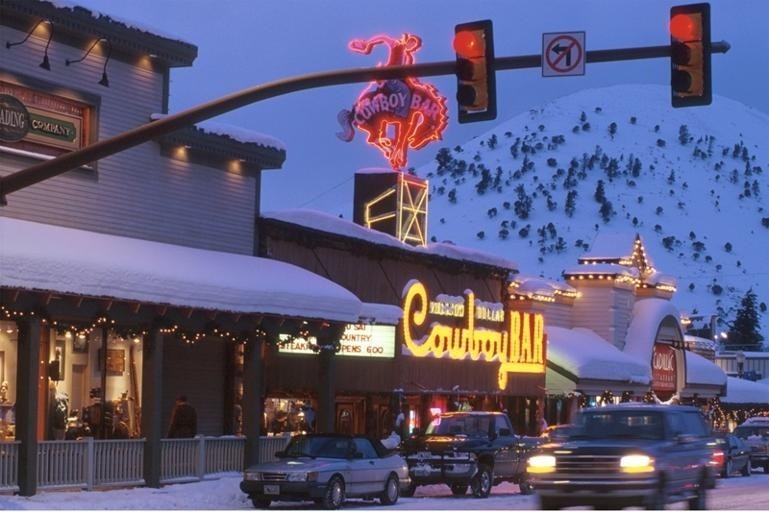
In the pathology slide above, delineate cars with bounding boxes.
[716,432,751,477]
[239,431,412,509]
[541,425,575,439]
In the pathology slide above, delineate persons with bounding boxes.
[165,393,199,439]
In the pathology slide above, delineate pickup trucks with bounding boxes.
[397,409,547,499]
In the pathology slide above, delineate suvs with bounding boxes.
[525,401,724,509]
[733,416,768,474]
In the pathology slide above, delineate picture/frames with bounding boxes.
[55,339,65,380]
[97,348,124,373]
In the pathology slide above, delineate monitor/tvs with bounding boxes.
[50,361,59,380]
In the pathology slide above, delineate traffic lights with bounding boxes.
[668,3,715,108]
[453,19,497,124]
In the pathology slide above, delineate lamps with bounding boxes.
[66,37,113,87]
[7,16,54,71]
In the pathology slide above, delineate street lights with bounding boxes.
[736,349,746,378]
